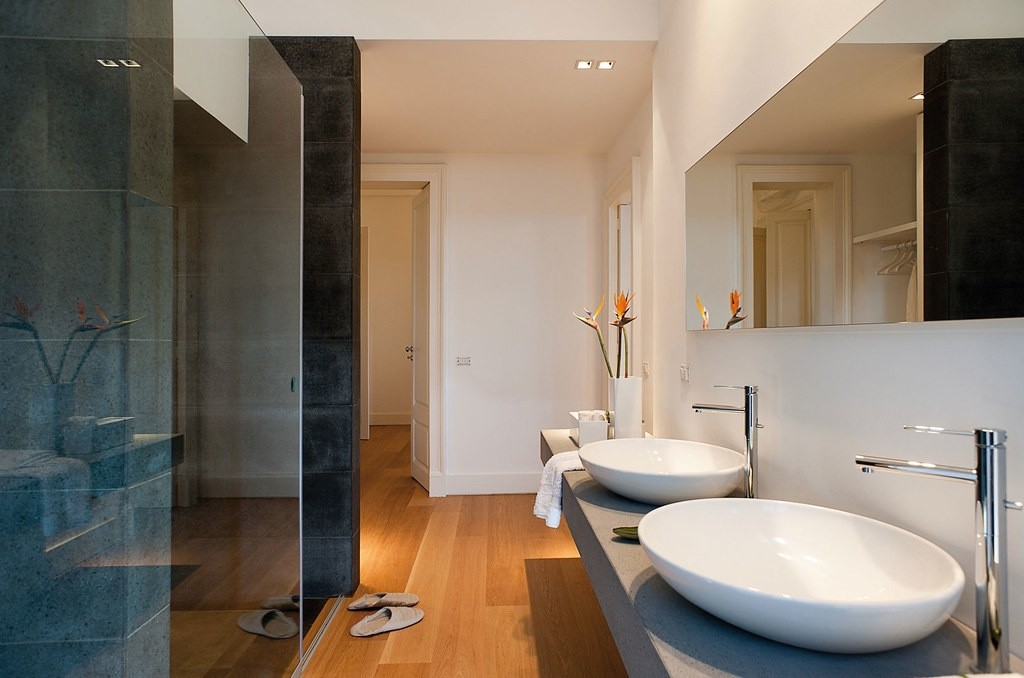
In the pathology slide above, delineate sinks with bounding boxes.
[636,496,967,653]
[577,436,746,506]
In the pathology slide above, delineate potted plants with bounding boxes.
[570,289,643,440]
[0,297,146,458]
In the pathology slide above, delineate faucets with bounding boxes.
[692,383,765,498]
[853,425,1020,674]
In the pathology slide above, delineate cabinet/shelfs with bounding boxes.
[848,111,923,322]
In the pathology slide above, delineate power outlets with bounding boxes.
[457,352,471,366]
[680,365,689,383]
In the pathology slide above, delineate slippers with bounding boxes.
[236,591,300,638]
[346,590,424,637]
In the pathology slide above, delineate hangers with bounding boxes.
[876,240,917,276]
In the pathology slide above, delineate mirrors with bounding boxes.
[684,0,1024,331]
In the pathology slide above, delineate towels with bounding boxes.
[532,449,585,530]
[1,446,91,539]
